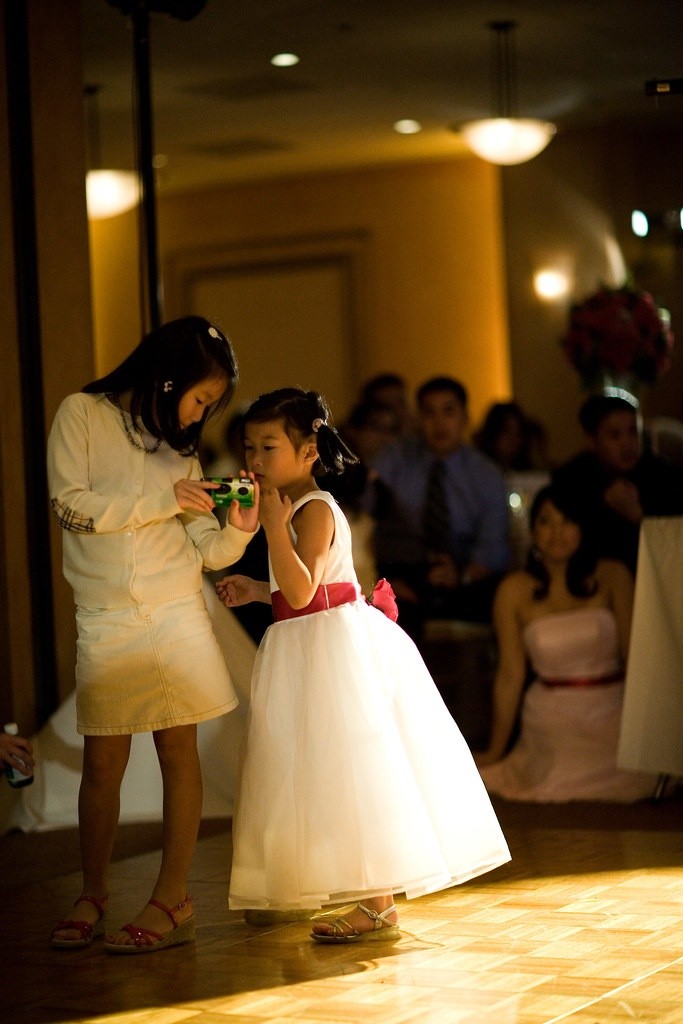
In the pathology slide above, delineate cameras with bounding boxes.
[199,476,255,508]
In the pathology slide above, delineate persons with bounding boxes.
[315,371,683,804]
[216,388,512,948]
[0,733,36,777]
[45,314,261,952]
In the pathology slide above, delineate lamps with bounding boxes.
[457,19,558,164]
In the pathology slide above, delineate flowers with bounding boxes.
[557,272,676,407]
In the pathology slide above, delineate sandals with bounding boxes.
[244,909,316,926]
[310,902,403,943]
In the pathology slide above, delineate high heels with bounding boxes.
[103,895,196,952]
[50,893,108,947]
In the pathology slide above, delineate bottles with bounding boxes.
[5,723,34,788]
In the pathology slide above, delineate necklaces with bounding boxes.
[114,396,163,455]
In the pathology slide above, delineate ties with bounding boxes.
[423,459,453,555]
